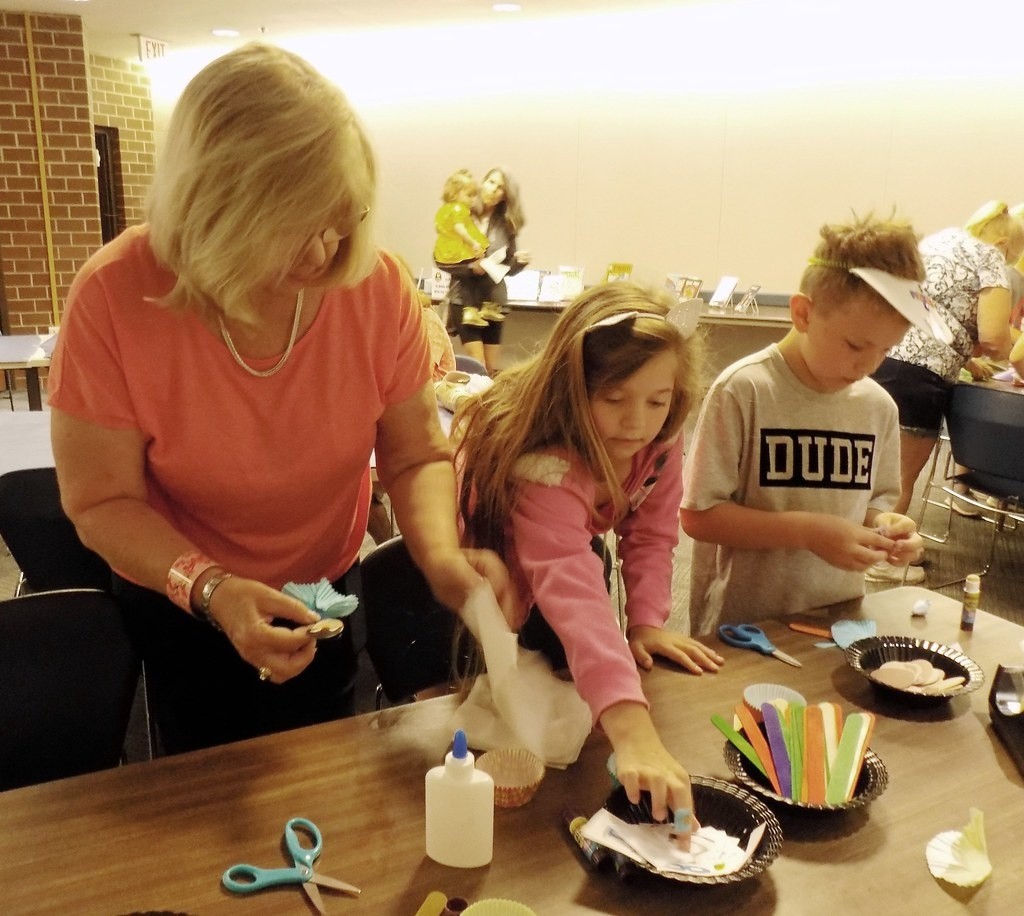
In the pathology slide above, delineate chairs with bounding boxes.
[1,466,118,599]
[358,535,443,709]
[0,584,143,792]
[916,382,1024,575]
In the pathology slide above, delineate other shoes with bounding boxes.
[461,306,489,326]
[480,301,506,322]
[970,488,1024,513]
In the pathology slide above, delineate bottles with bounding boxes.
[424,729,495,868]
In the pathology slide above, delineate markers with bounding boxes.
[562,805,631,882]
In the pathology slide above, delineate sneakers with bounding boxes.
[864,564,925,585]
[944,490,978,516]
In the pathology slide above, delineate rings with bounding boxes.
[259,667,272,682]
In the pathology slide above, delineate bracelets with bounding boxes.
[166,550,223,624]
[202,573,232,632]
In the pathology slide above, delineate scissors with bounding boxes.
[222,815,362,916]
[718,622,802,668]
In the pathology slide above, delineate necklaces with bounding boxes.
[218,287,305,377]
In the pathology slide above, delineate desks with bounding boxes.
[1,335,59,411]
[0,585,1024,916]
[505,298,793,337]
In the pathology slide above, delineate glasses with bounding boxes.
[322,204,370,243]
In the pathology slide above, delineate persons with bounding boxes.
[679,218,919,636]
[449,284,727,837]
[871,205,1024,584]
[48,49,526,755]
[434,167,527,379]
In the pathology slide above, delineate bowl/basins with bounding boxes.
[743,684,807,725]
[602,774,784,885]
[475,748,545,808]
[458,899,536,916]
[844,635,984,700]
[723,726,888,813]
